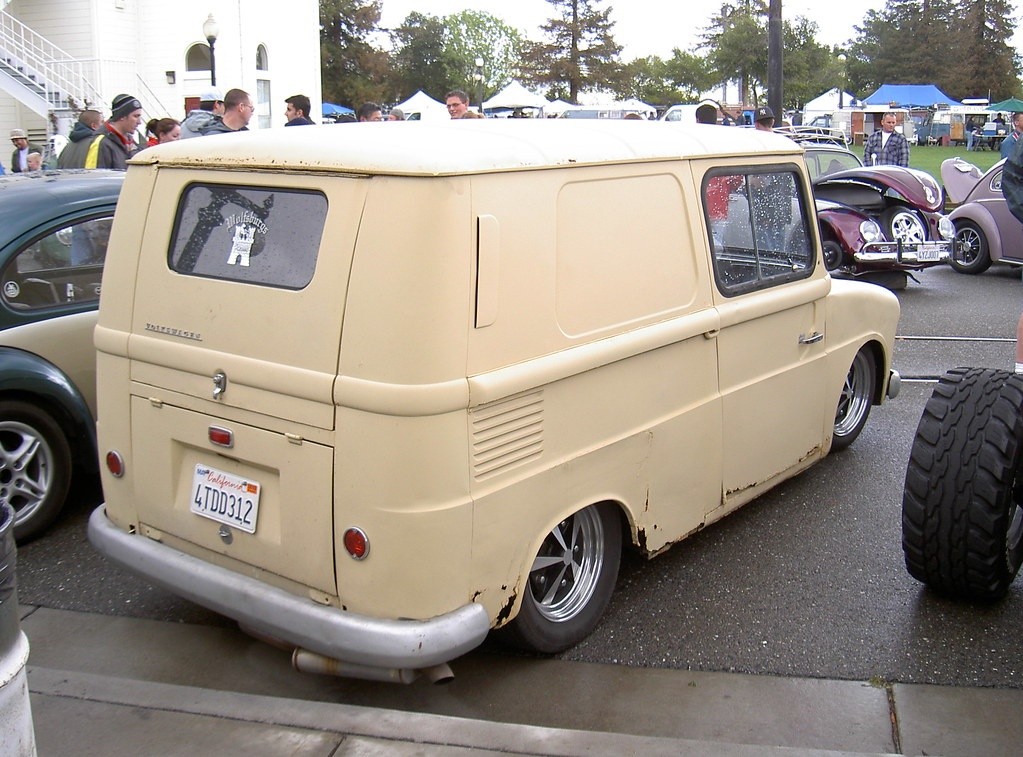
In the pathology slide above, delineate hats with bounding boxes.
[199,86,224,101]
[111,94,143,122]
[754,107,775,123]
[8,130,29,140]
[390,109,405,120]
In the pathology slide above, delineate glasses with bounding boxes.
[244,105,254,112]
[446,102,465,108]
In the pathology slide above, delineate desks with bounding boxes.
[973,133,1010,152]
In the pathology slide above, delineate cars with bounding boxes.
[941,156,1023,276]
[724,124,963,290]
[0,169,130,545]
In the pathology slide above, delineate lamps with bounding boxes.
[163,71,177,85]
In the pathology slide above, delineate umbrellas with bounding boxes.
[986,95,1023,131]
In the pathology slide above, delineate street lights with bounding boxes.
[200,11,221,86]
[837,54,848,109]
[471,56,485,114]
[173,188,276,274]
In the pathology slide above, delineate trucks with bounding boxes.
[806,105,1016,146]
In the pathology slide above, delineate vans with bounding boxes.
[85,116,904,687]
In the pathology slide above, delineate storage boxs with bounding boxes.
[984,122,998,135]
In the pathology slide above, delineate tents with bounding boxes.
[538,97,656,120]
[866,84,963,124]
[322,102,353,121]
[393,89,447,110]
[482,78,548,118]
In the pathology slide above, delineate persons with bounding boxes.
[862,113,909,169]
[284,93,316,126]
[56,87,255,266]
[694,104,743,254]
[782,108,803,125]
[445,90,478,119]
[1000,112,1023,159]
[721,108,752,126]
[990,113,1005,152]
[335,100,405,124]
[477,109,484,119]
[656,111,669,121]
[9,128,48,171]
[625,113,643,119]
[1001,133,1023,374]
[965,116,985,152]
[494,108,530,118]
[745,104,793,252]
[648,112,655,120]
